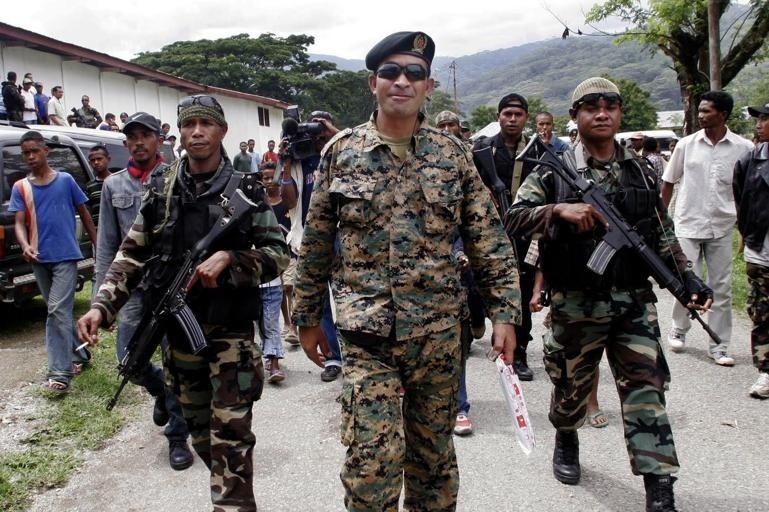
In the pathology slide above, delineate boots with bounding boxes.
[642,475,680,512]
[552,429,581,485]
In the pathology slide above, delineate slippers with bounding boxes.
[279,327,300,344]
[264,358,284,382]
[51,378,72,393]
[70,359,89,375]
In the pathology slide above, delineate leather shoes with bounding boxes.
[152,390,171,425]
[168,436,195,470]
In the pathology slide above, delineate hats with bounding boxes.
[498,93,528,111]
[123,112,159,135]
[628,131,645,140]
[571,77,622,107]
[365,31,435,71]
[435,110,471,130]
[746,104,769,119]
[177,95,228,128]
[8,72,43,87]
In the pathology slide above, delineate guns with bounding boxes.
[106,187,258,412]
[516,134,721,344]
[72,107,92,128]
[474,146,526,274]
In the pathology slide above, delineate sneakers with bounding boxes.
[749,371,769,398]
[453,411,474,436]
[668,326,686,353]
[709,346,734,366]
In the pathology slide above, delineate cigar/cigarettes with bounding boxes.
[701,307,715,314]
[75,340,89,354]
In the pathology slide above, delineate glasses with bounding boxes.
[377,62,427,82]
[573,92,620,102]
[178,96,218,110]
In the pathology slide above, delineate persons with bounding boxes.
[75,94,290,511]
[502,73,715,512]
[0,66,681,440]
[87,111,195,471]
[290,28,527,510]
[733,99,769,401]
[657,88,756,365]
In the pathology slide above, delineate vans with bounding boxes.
[1,123,177,312]
[612,128,681,156]
[0,126,105,301]
